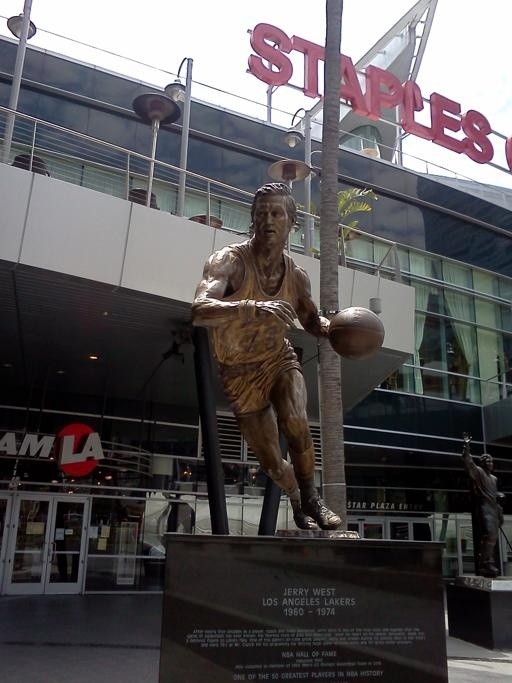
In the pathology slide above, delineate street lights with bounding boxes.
[166,56,194,218]
[127,93,182,208]
[265,155,312,205]
[1,1,37,164]
[286,107,315,255]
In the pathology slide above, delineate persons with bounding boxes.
[187,183,343,528]
[462,428,506,580]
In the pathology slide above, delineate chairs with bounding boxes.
[10,154,51,177]
[129,188,222,228]
